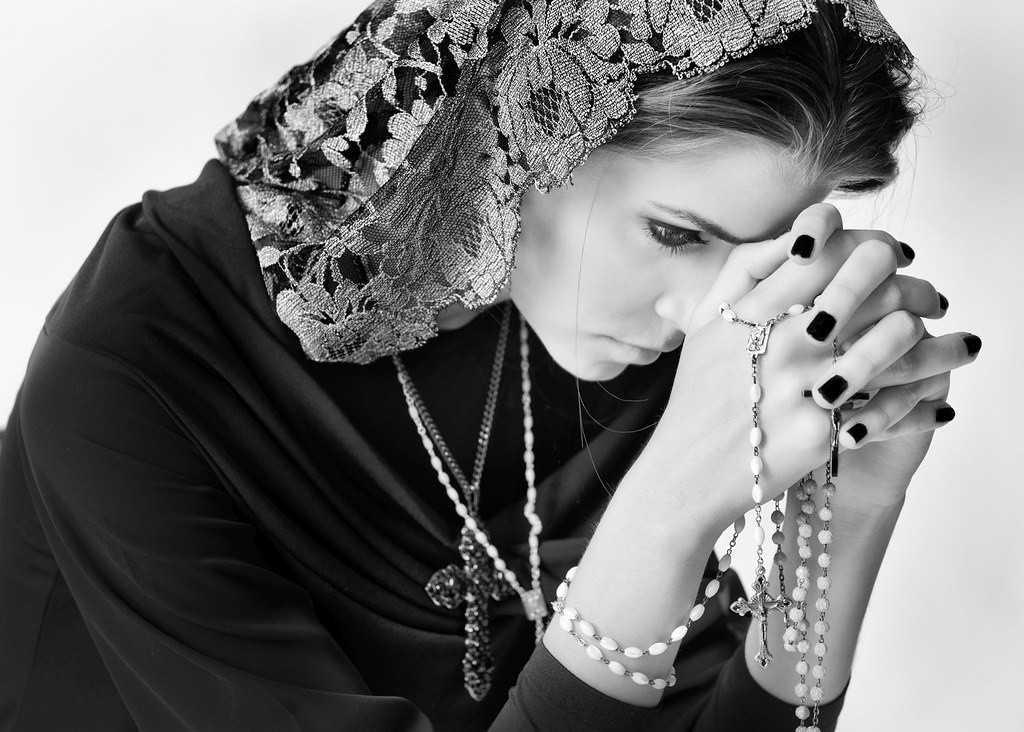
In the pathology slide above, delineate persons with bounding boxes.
[0,0,983,732]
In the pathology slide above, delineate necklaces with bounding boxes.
[389,303,848,732]
